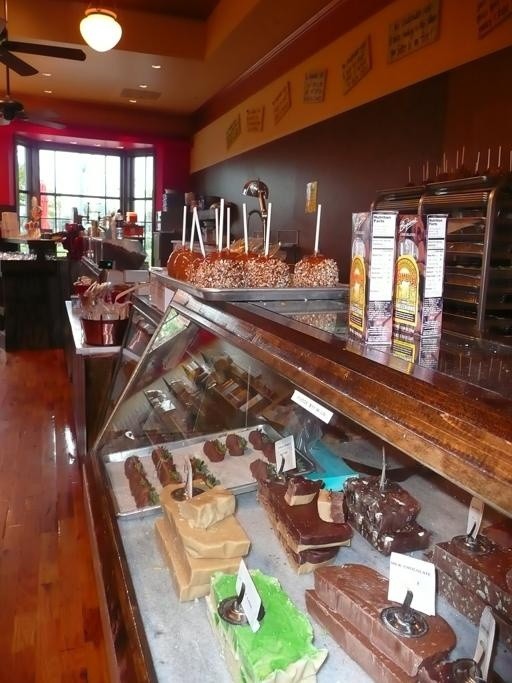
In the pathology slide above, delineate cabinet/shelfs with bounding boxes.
[370,174,511,341]
[83,290,510,683]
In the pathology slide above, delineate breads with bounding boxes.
[151,352,277,435]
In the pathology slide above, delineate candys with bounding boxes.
[166,199,343,289]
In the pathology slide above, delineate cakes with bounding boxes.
[154,479,250,603]
[304,563,457,683]
[420,533,512,650]
[205,570,318,683]
[255,476,353,575]
[338,476,432,557]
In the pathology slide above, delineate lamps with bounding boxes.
[79,0,123,52]
[0,66,24,119]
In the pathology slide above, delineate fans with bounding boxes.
[0,0,87,77]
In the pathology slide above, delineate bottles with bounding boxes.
[115,209,124,240]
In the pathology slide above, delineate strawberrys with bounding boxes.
[124,428,277,510]
